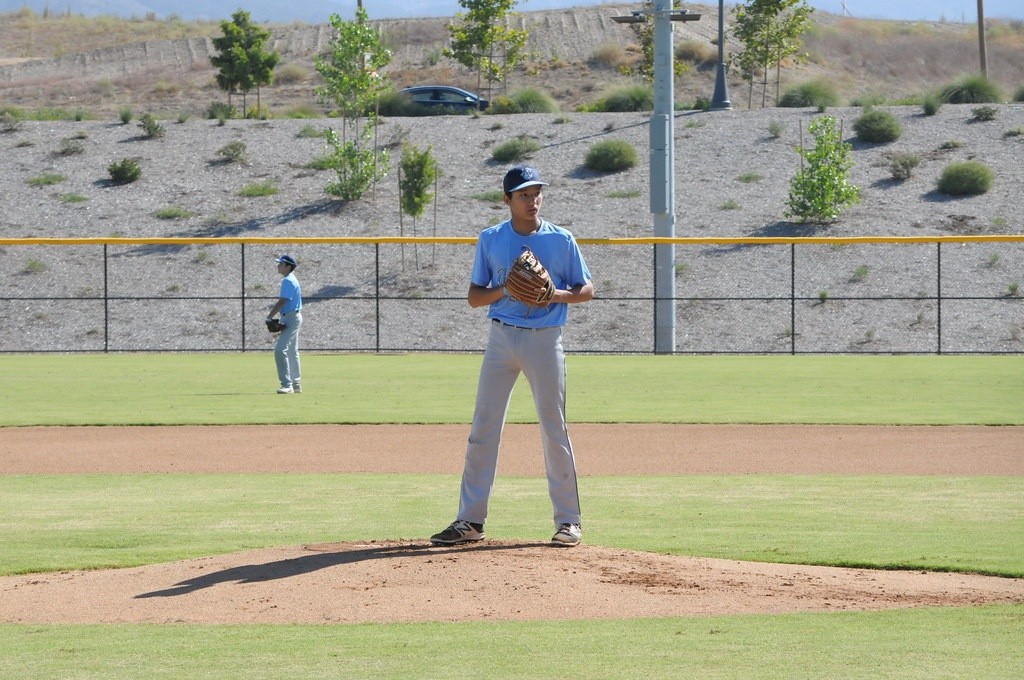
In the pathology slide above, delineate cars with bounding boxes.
[360,85,489,117]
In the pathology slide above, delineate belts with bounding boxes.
[283,310,300,316]
[492,318,532,330]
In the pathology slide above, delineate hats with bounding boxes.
[503,164,549,194]
[274,255,295,266]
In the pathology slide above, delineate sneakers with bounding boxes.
[429,519,485,543]
[276,384,295,394]
[293,383,302,392]
[551,522,582,546]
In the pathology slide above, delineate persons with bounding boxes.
[430,166,595,547]
[264,255,303,394]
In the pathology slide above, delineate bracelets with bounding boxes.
[503,287,510,298]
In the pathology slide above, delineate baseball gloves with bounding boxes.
[504,249,556,308]
[265,319,287,333]
